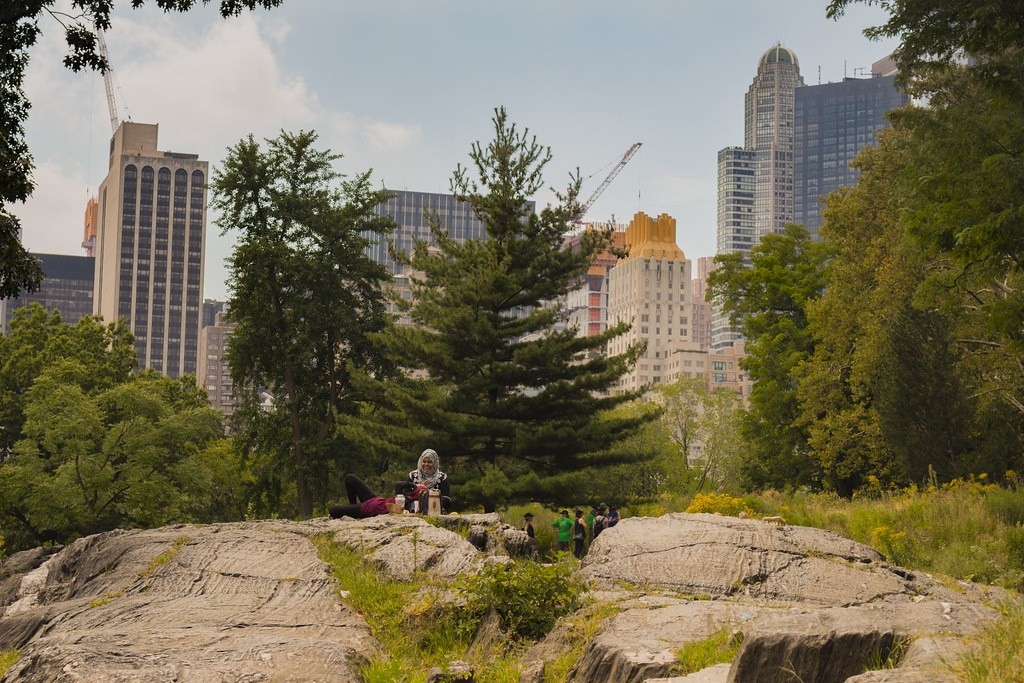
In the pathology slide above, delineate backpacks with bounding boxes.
[594,517,605,536]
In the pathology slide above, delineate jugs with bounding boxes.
[428,489,441,515]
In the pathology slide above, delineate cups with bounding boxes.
[395,494,405,510]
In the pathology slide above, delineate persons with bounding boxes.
[402,449,451,515]
[524,513,534,537]
[573,510,588,558]
[330,474,431,519]
[551,510,575,552]
[587,503,618,545]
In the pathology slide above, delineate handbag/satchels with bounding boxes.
[574,532,584,541]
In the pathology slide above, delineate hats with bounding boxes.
[524,513,534,517]
[575,510,584,517]
[597,503,608,510]
[560,510,569,514]
